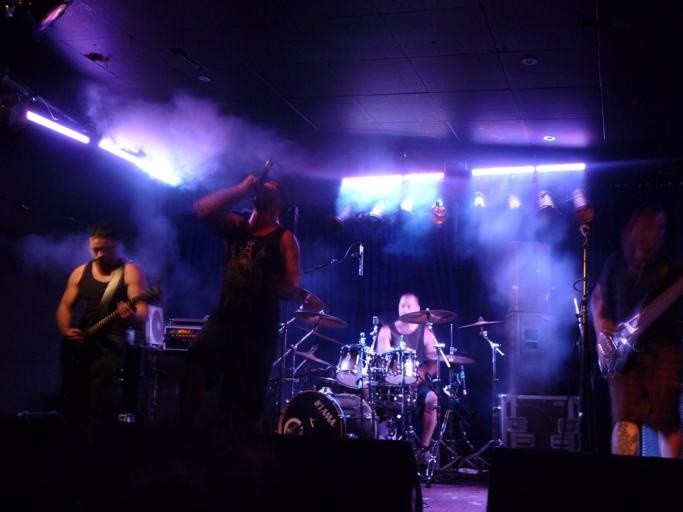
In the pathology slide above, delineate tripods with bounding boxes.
[397,386,422,482]
[455,331,507,485]
[425,369,481,488]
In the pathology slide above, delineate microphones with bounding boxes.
[359,244,364,278]
[250,160,273,194]
[580,224,590,237]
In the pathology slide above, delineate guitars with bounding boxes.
[597,278,683,373]
[60,286,160,367]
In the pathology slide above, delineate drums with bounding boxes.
[279,388,378,440]
[382,348,420,385]
[337,344,379,390]
[368,385,420,414]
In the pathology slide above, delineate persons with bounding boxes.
[375,293,441,448]
[589,202,683,460]
[182,160,304,420]
[55,224,149,411]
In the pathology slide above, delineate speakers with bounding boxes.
[487,448,683,512]
[0,413,271,512]
[267,440,422,512]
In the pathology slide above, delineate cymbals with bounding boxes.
[294,288,328,307]
[298,324,345,347]
[400,310,457,326]
[426,352,475,364]
[459,321,501,331]
[291,311,350,329]
[290,350,333,368]
